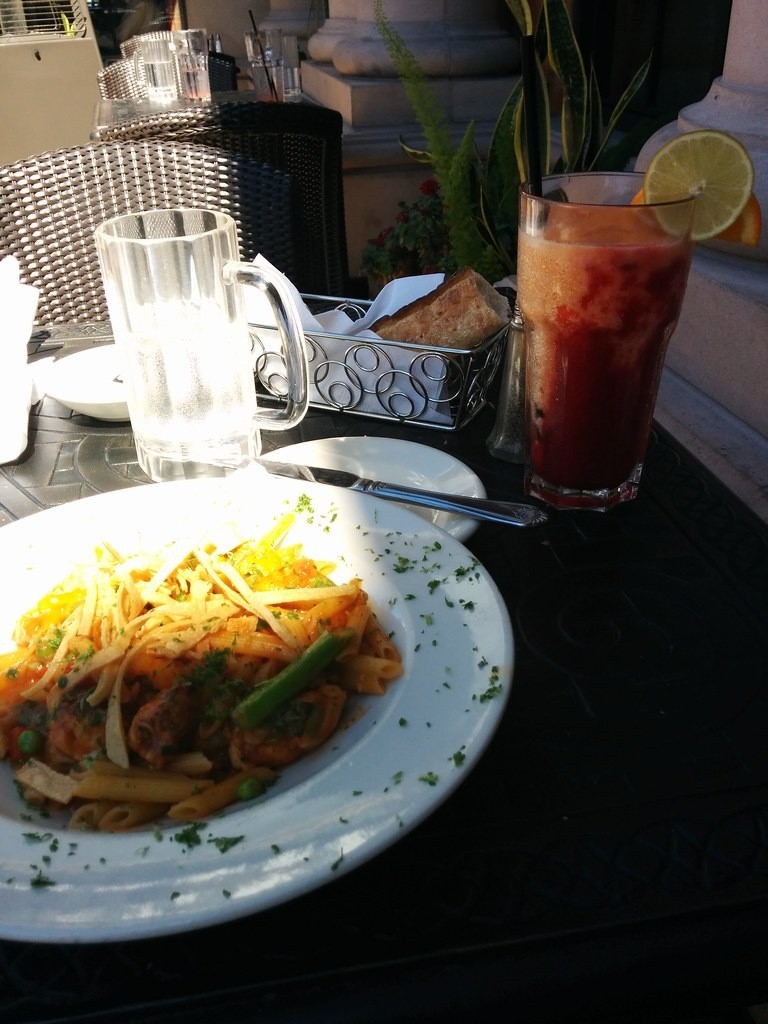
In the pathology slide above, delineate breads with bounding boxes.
[365,267,510,359]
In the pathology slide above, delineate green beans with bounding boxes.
[17,729,43,753]
[237,776,266,800]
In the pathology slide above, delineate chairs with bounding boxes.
[96,50,238,100]
[100,101,350,314]
[120,29,208,58]
[0,140,297,333]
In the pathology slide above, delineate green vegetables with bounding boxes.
[0,489,503,905]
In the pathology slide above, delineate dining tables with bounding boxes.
[89,90,325,146]
[0,287,768,1024]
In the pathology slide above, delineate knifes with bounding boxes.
[255,455,539,529]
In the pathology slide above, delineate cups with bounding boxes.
[133,36,178,107]
[94,207,309,482]
[243,28,285,102]
[515,171,696,514]
[283,35,303,103]
[173,29,211,103]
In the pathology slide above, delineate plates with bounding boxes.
[0,475,518,945]
[43,340,132,424]
[227,436,486,546]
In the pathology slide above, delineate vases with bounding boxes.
[366,266,407,301]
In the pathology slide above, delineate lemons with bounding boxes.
[643,130,754,239]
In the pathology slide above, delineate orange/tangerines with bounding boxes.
[629,187,761,247]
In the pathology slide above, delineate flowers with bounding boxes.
[362,180,449,275]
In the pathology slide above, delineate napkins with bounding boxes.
[190,252,454,426]
[0,255,39,464]
